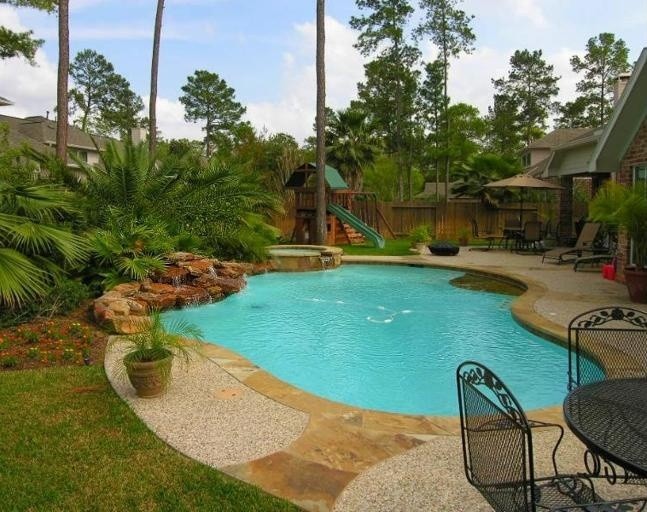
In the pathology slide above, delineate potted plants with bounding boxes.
[409,224,432,254]
[108,306,206,399]
[456,228,471,246]
[585,179,647,303]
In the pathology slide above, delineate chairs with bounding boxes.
[457,361,647,512]
[541,220,602,263]
[568,306,647,485]
[471,219,552,256]
[573,255,614,271]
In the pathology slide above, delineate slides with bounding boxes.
[326,203,385,248]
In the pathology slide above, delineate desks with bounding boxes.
[586,248,609,268]
[563,378,647,478]
